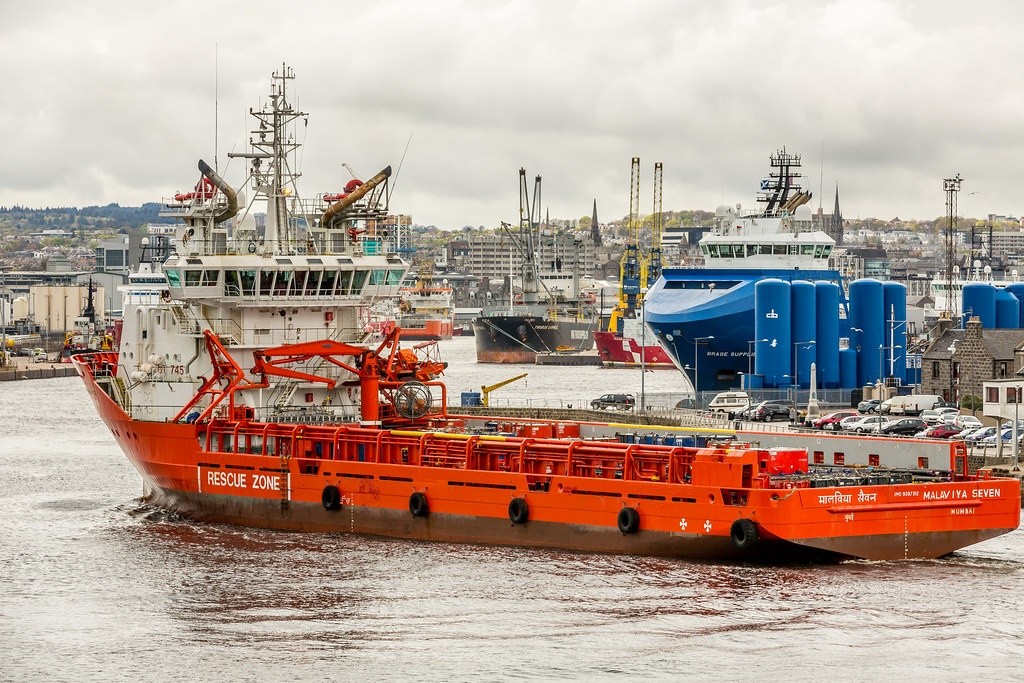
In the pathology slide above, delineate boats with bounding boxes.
[360,123,1024,413]
[59,273,116,363]
[69,56,1024,564]
[0,296,42,352]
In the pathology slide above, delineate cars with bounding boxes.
[18,347,30,357]
[731,385,1023,449]
[8,350,17,356]
[30,349,35,356]
[37,353,48,361]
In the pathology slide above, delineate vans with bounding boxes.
[34,348,44,357]
